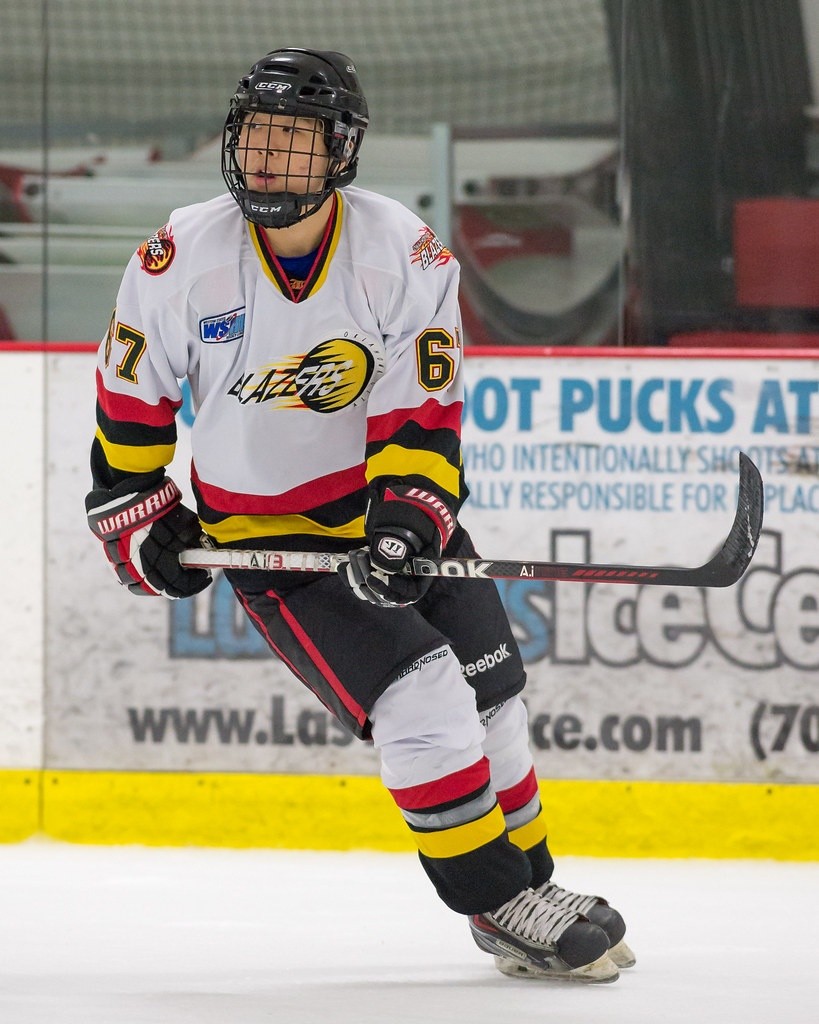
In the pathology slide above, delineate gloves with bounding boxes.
[85,466,213,601]
[336,476,459,609]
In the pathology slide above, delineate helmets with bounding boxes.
[220,46,370,229]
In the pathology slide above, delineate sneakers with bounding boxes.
[467,879,636,984]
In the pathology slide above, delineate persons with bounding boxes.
[88,49,635,987]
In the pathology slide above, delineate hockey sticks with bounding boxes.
[176,448,767,589]
